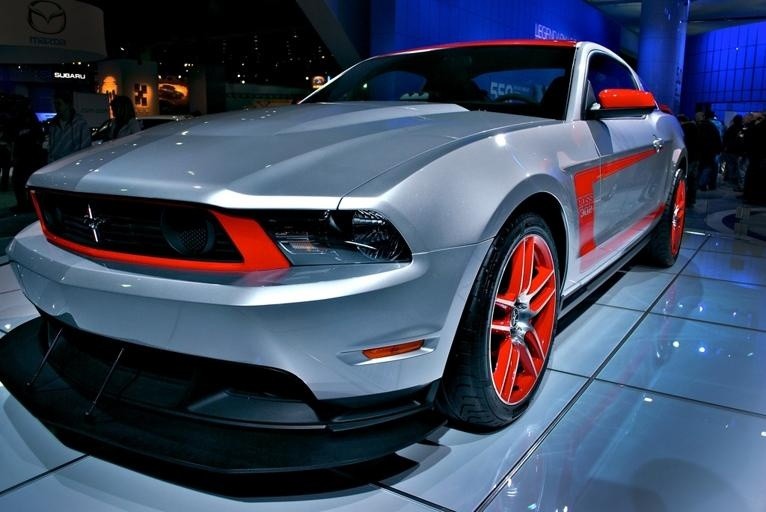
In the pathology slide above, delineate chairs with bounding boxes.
[420,78,486,101]
[540,76,570,107]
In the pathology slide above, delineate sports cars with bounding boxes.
[0,36,691,500]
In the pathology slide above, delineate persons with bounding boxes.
[45,87,97,164]
[0,92,43,214]
[676,110,765,208]
[103,96,141,141]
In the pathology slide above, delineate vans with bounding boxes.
[82,114,186,150]
